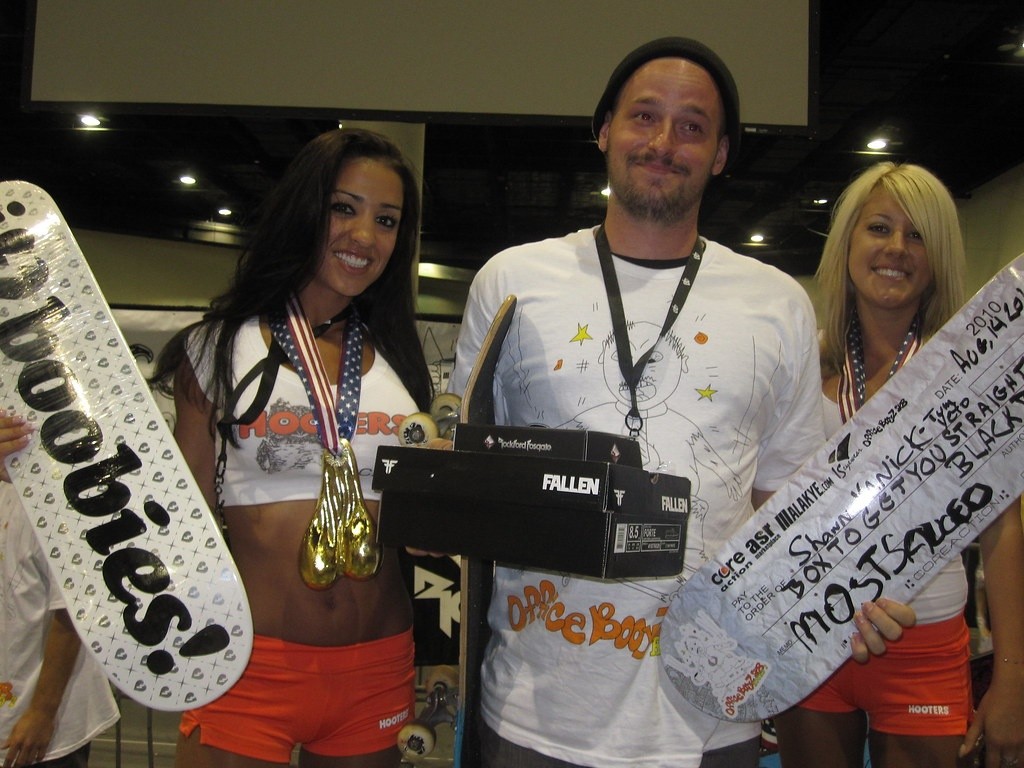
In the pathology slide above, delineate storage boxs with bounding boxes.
[372,424,691,578]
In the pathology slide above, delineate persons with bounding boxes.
[778,164,1024,768]
[449,37,915,768]
[0,129,448,768]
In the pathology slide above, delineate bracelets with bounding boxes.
[994,650,1024,666]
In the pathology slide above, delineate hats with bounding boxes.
[592,37,742,155]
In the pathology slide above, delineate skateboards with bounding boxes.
[651,234,1024,734]
[372,289,524,768]
[0,172,259,717]
